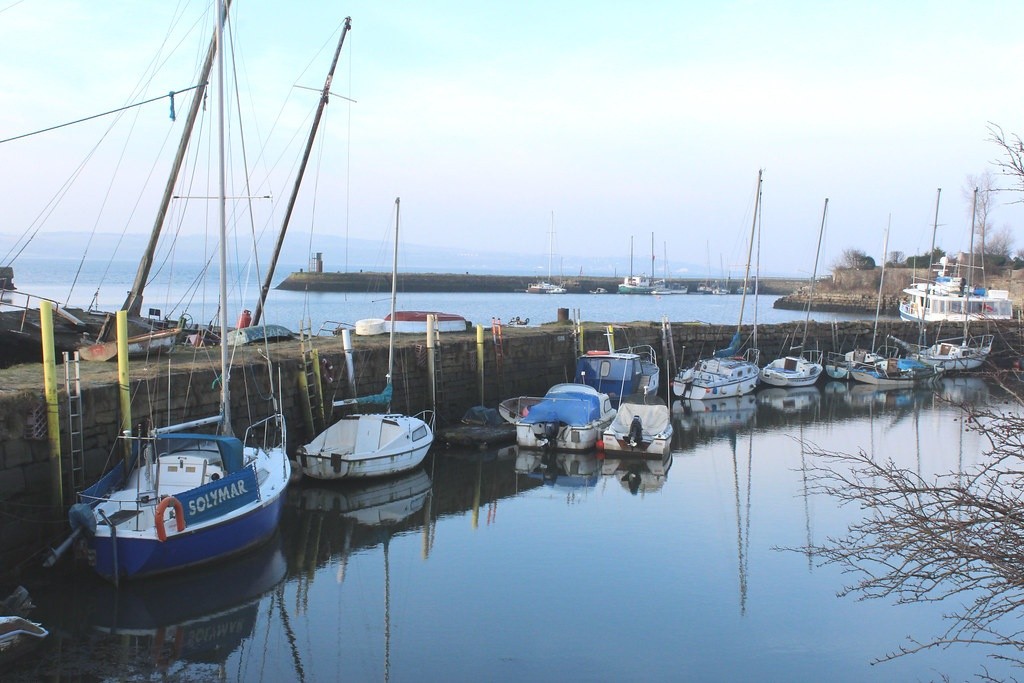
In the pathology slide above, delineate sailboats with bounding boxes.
[1,0,356,366]
[672,396,762,617]
[295,460,433,683]
[825,213,896,380]
[294,197,438,480]
[68,0,292,581]
[755,382,822,573]
[67,537,308,683]
[674,165,765,402]
[757,197,830,387]
[524,208,757,297]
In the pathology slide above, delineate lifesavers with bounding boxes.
[587,350,610,356]
[155,496,186,543]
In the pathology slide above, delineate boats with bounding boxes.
[597,449,673,495]
[850,355,947,389]
[919,333,994,375]
[570,344,660,414]
[498,395,543,425]
[604,402,674,458]
[513,451,601,491]
[516,383,618,453]
[440,401,519,453]
[899,187,1014,333]
